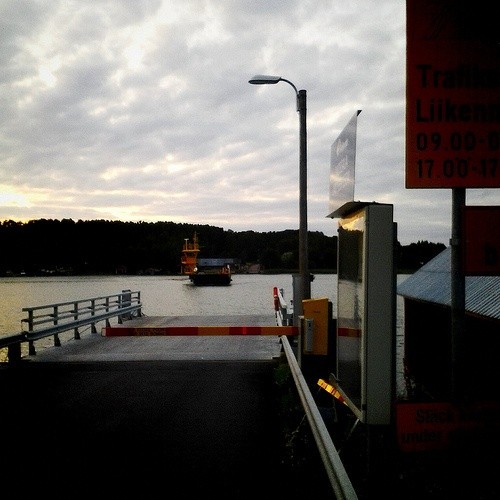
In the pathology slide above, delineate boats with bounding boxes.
[188,258,232,286]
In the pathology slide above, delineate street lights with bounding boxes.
[248,72,309,326]
